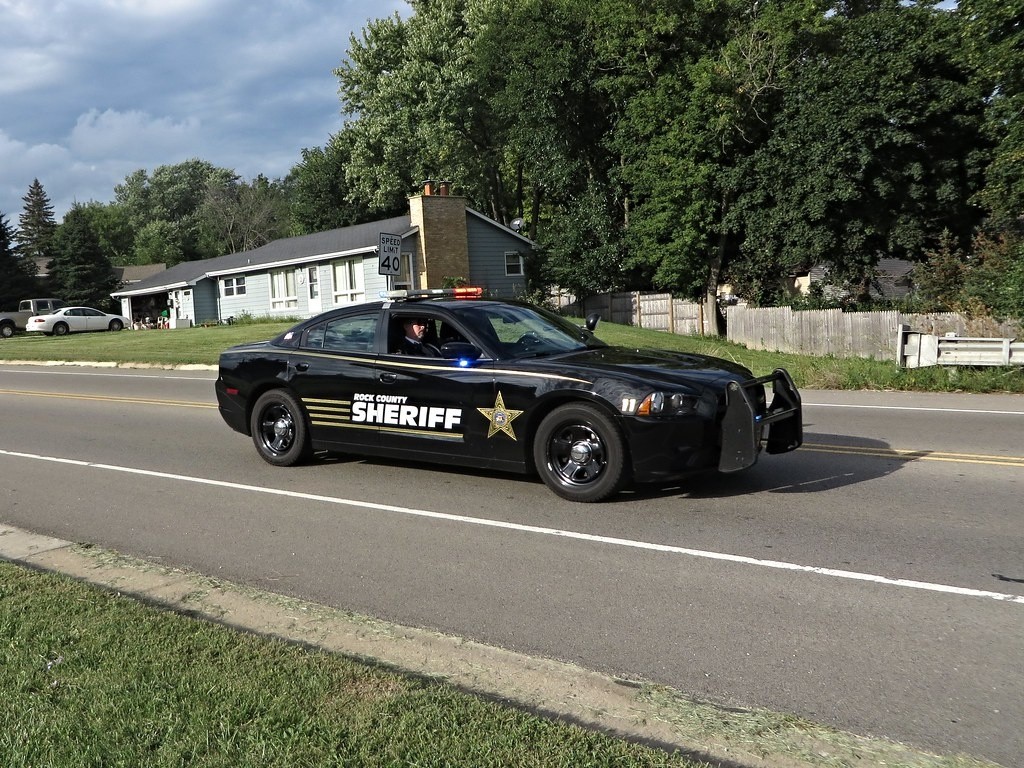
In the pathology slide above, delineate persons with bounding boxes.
[435,316,481,361]
[391,316,442,359]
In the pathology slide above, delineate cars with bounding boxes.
[26,306,131,336]
[214,286,803,504]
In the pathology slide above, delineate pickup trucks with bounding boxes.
[0,299,70,337]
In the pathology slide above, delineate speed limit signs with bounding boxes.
[378,233,401,275]
